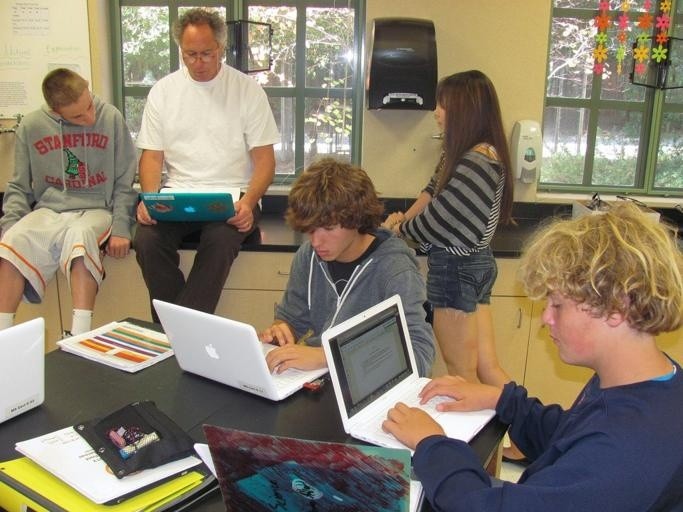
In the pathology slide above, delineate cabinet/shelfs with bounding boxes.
[212,252,681,450]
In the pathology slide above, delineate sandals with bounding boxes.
[502,456,532,466]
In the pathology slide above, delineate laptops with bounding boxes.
[152,299,330,401]
[321,295,498,458]
[202,423,426,512]
[1,317,45,425]
[140,187,241,223]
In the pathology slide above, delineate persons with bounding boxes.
[135,9,281,324]
[258,157,437,379]
[0,68,138,336]
[382,202,683,512]
[380,70,532,466]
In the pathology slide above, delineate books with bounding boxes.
[0,425,221,512]
[55,321,175,373]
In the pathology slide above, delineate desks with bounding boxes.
[0,316,509,511]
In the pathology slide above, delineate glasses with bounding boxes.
[182,49,215,65]
